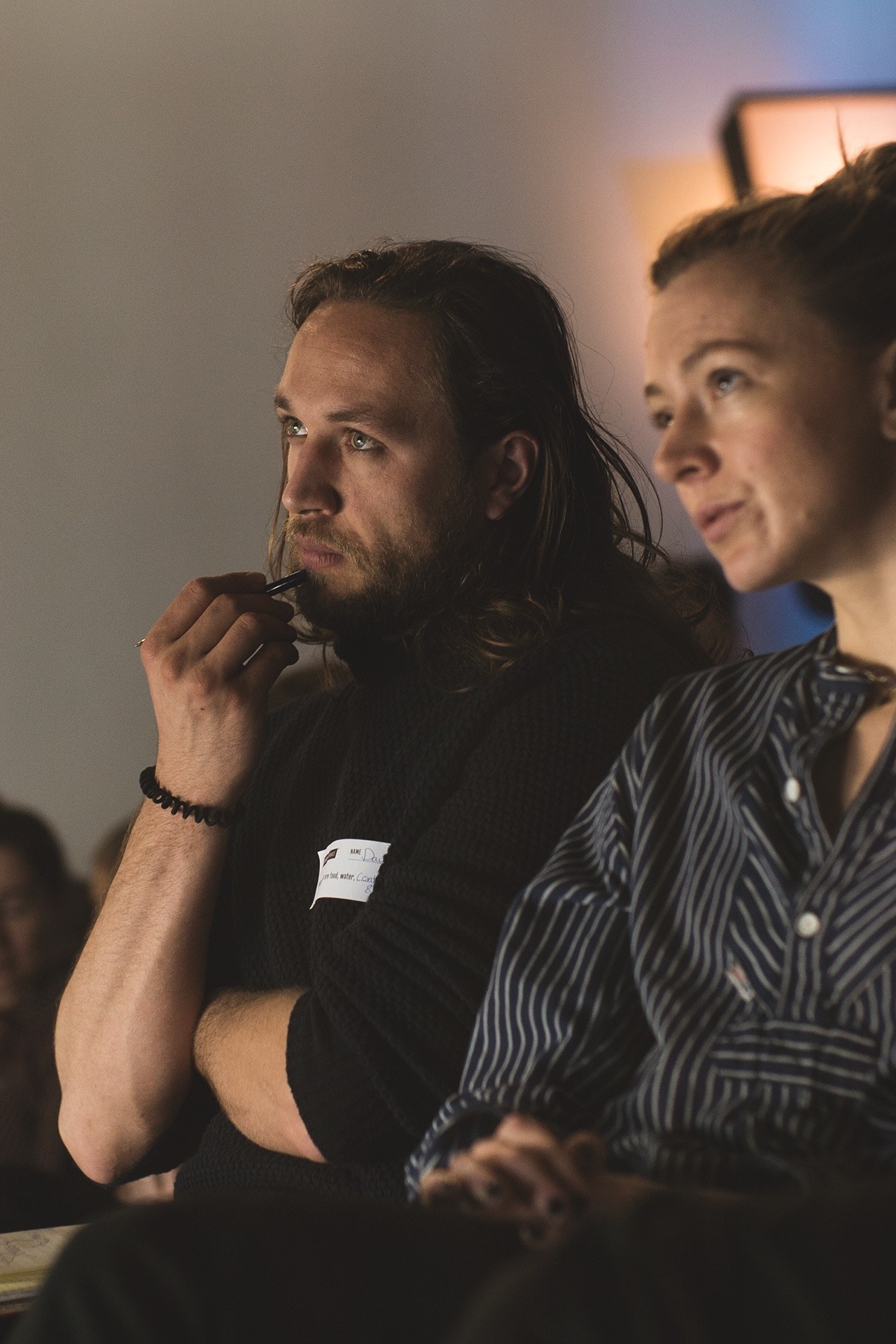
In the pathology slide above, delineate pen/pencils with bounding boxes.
[132,567,313,653]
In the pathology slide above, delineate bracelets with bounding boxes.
[139,766,246,828]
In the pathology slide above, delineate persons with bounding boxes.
[0,802,78,1233]
[90,820,181,1203]
[53,239,699,1182]
[12,140,894,1343]
